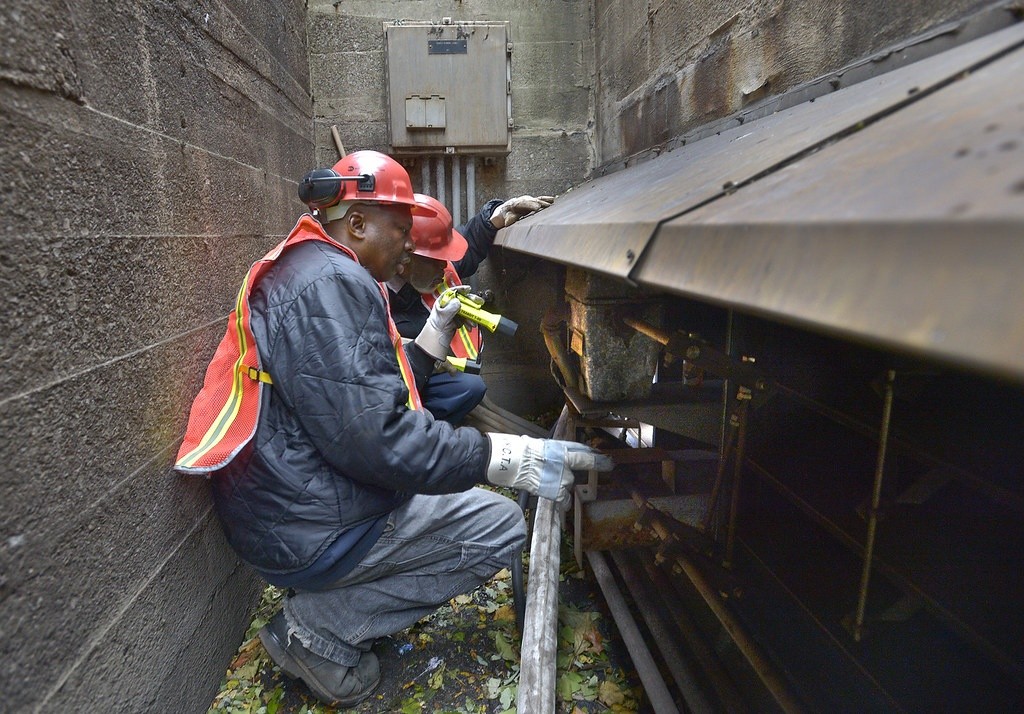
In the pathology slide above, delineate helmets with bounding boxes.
[409,194,469,262]
[316,150,439,218]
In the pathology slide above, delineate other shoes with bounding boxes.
[258,607,381,708]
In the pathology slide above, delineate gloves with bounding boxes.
[489,196,555,230]
[414,285,484,363]
[483,432,616,512]
[400,337,458,375]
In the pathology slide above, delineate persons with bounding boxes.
[174,151,615,708]
[380,194,554,422]
